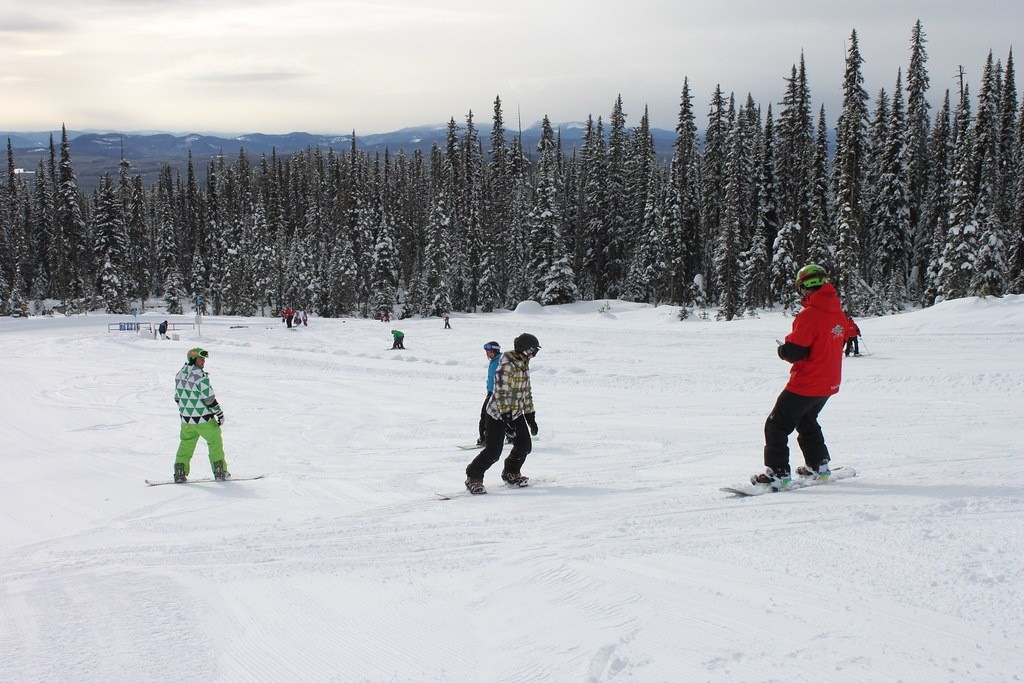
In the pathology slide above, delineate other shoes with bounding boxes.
[465,477,486,494]
[476,435,486,444]
[173,463,188,482]
[213,461,232,482]
[501,471,528,489]
[505,434,515,441]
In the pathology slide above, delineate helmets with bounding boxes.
[188,346,207,362]
[483,341,501,357]
[514,333,541,359]
[795,265,829,288]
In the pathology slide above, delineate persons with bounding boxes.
[444,314,450,328]
[284,307,307,328]
[845,318,861,357]
[173,347,232,482]
[392,330,404,349]
[750,264,851,488]
[464,333,542,495]
[159,320,168,340]
[381,312,389,322]
[477,341,512,444]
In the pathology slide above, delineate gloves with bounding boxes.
[527,413,538,436]
[500,411,516,443]
[214,410,224,427]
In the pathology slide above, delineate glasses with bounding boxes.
[198,349,208,357]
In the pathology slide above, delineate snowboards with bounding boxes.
[144,474,266,487]
[455,436,541,451]
[433,472,557,499]
[717,465,857,496]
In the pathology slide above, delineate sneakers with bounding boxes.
[751,468,792,484]
[795,464,832,479]
